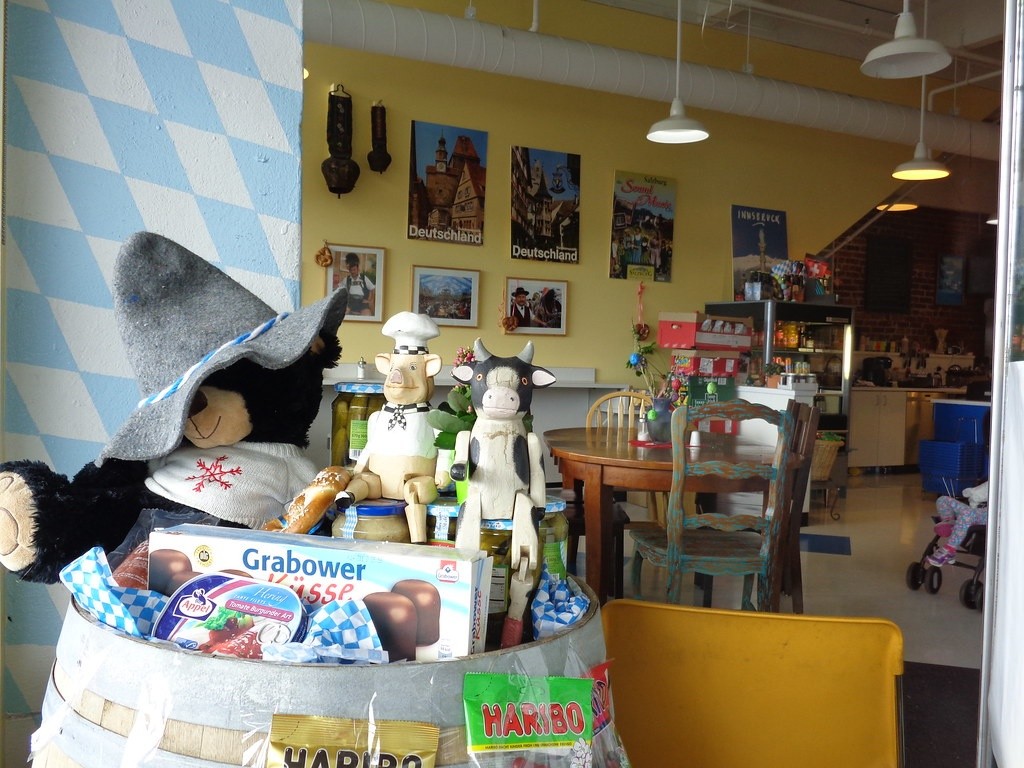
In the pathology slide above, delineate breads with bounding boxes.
[262,465,352,536]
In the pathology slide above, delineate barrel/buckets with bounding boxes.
[931,398,991,448]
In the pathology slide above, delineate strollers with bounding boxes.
[906,475,983,611]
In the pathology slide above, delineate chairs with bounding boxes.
[600,597,907,768]
[700,399,822,616]
[629,398,796,607]
[585,390,669,522]
[547,502,630,599]
[546,487,617,504]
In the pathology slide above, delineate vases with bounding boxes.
[646,398,674,442]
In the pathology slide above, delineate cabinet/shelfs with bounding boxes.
[845,391,949,465]
[704,301,856,499]
[929,398,991,480]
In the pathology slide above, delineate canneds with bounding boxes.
[330,382,387,468]
[331,498,409,543]
[780,357,817,386]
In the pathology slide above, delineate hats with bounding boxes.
[512,287,529,297]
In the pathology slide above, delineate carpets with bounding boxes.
[901,659,981,768]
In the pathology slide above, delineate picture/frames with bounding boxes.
[504,276,569,336]
[323,242,386,323]
[409,264,481,329]
[934,253,967,307]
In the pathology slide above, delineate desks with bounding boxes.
[542,427,807,610]
[305,361,630,484]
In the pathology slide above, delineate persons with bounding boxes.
[510,288,562,328]
[924,496,988,567]
[343,253,375,316]
[611,226,672,275]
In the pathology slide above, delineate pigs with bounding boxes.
[335,353,443,548]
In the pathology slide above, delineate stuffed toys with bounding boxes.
[0,307,341,585]
[963,481,988,508]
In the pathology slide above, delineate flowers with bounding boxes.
[452,346,479,367]
[647,355,692,422]
[625,342,658,377]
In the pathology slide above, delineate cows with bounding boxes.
[449,337,556,648]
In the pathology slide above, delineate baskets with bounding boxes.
[811,436,844,481]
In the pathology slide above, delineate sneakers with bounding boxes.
[933,520,955,536]
[924,547,955,567]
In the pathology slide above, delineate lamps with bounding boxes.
[890,0,952,182]
[876,196,918,212]
[858,0,953,80]
[985,211,998,226]
[645,0,711,145]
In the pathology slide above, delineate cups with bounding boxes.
[785,358,792,373]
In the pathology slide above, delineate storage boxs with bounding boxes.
[815,395,841,416]
[658,309,754,353]
[670,348,742,376]
[672,375,736,407]
[918,437,982,503]
[693,418,738,435]
[145,522,496,664]
